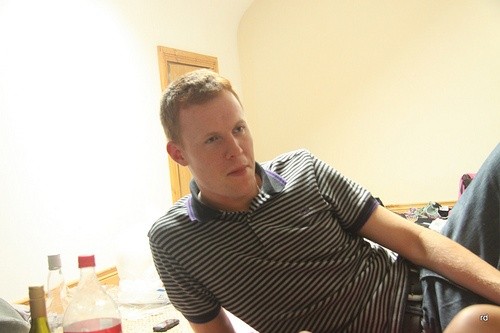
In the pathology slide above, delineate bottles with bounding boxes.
[28,285,52,333]
[27,254,77,333]
[63,255,122,333]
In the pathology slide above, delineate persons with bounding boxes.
[147,68,500,333]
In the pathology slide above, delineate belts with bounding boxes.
[401,260,423,332]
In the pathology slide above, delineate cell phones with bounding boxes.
[153,319,179,332]
